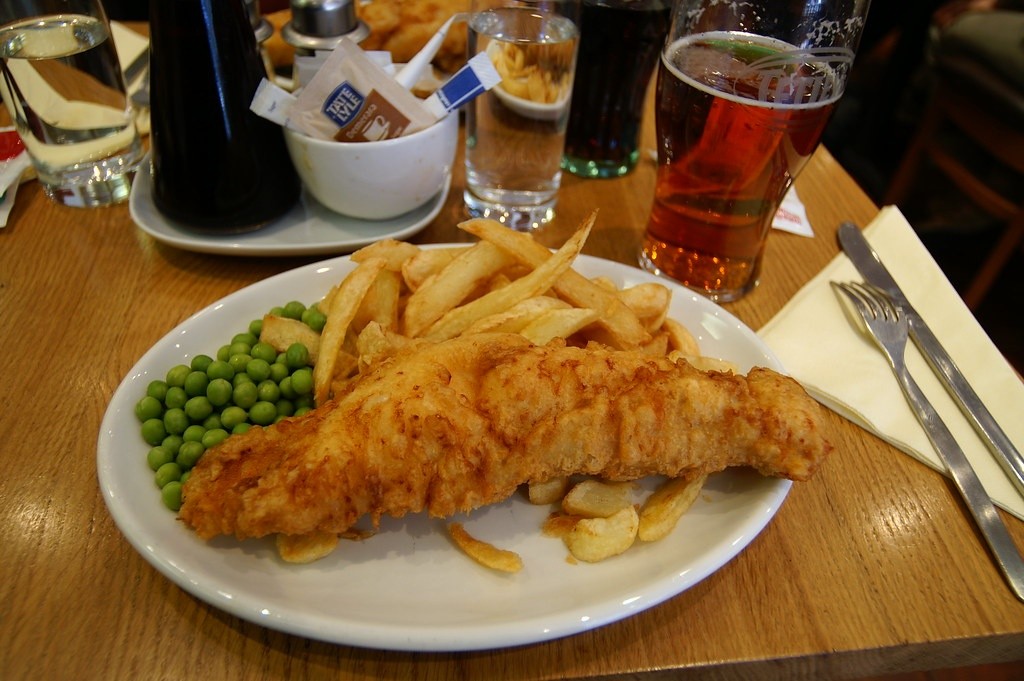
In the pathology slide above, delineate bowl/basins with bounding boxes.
[283,107,459,221]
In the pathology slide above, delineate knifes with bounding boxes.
[834,220,1024,499]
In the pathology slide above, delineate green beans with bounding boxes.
[135,299,328,512]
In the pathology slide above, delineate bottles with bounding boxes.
[148,0,302,237]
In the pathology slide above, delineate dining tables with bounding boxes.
[1,20,1024,681]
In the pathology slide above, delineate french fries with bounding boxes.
[482,35,570,104]
[258,207,742,573]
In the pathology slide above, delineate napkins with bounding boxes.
[758,205,1024,520]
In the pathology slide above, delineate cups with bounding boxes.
[461,5,579,233]
[637,0,872,305]
[554,0,675,179]
[0,0,145,209]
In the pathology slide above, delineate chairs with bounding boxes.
[888,0,1024,309]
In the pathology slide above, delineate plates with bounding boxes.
[95,242,795,654]
[129,144,452,255]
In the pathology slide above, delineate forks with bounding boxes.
[830,281,1024,604]
